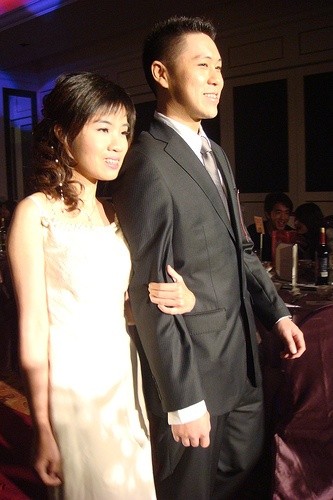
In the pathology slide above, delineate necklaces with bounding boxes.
[81,200,97,222]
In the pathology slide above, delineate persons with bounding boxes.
[8,71,196,500]
[112,17,333,500]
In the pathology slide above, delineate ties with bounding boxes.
[199,136,231,222]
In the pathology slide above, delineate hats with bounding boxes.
[290,202,323,224]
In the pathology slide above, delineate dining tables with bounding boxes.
[254,259,333,441]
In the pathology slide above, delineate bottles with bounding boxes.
[258,233,264,250]
[315,227,329,286]
[275,231,297,282]
